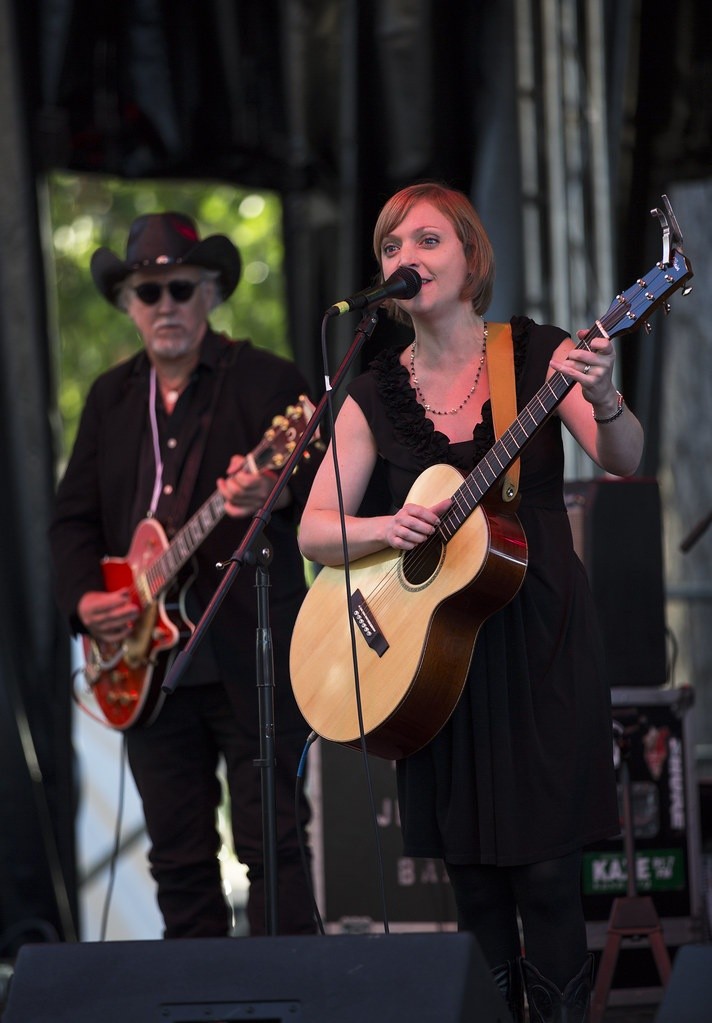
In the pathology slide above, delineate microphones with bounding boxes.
[325,268,422,318]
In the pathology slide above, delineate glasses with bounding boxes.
[125,276,208,306]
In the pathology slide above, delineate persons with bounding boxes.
[295,183,645,1023]
[46,210,334,941]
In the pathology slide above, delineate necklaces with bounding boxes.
[410,321,488,416]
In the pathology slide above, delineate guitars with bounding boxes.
[78,392,325,737]
[284,190,697,763]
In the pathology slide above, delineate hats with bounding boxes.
[90,213,243,313]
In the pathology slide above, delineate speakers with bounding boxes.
[564,479,667,688]
[0,930,517,1023]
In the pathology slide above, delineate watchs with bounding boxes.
[592,390,625,424]
